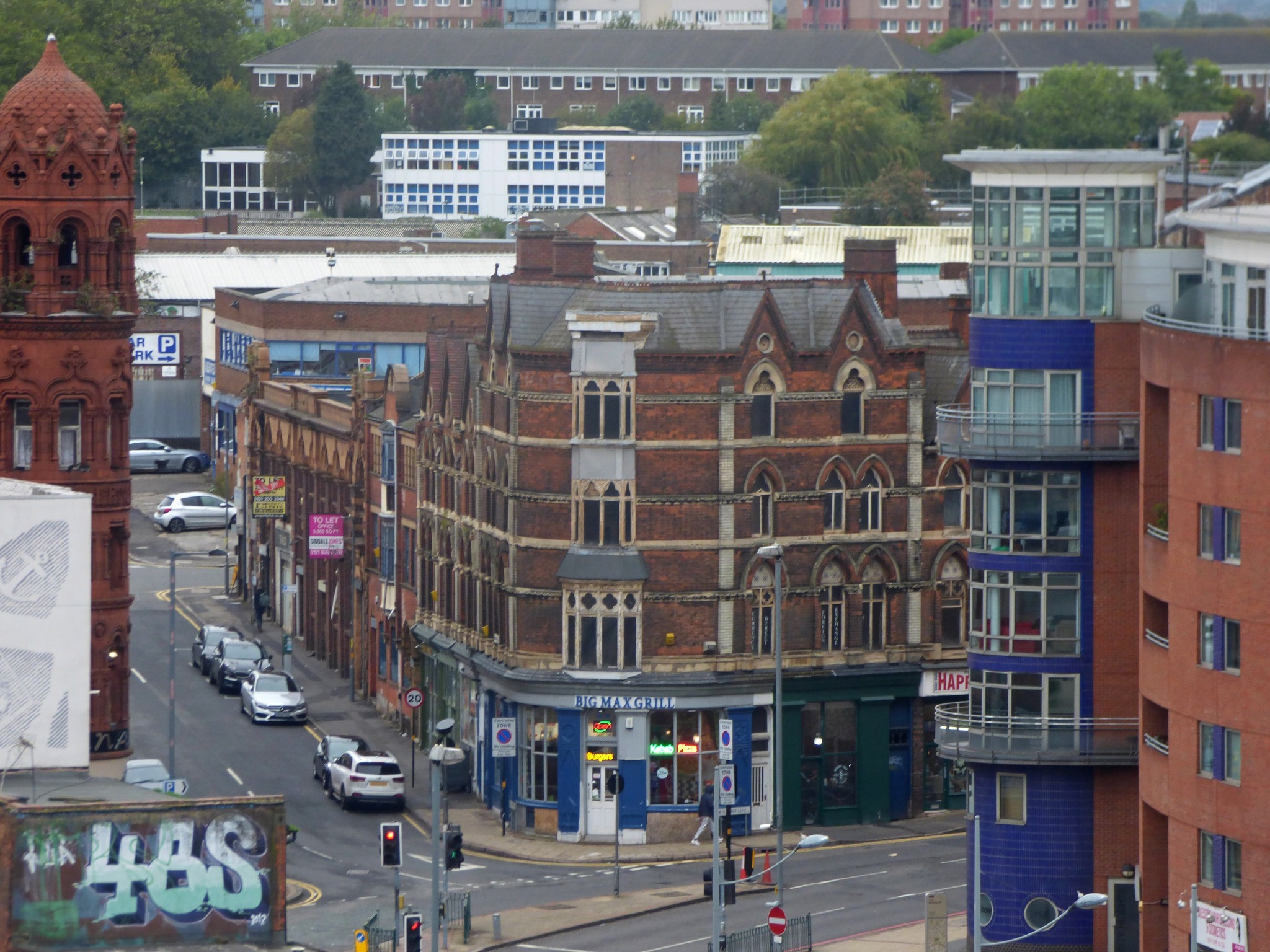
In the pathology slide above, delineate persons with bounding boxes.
[684,791,693,804]
[250,584,269,633]
[690,785,724,846]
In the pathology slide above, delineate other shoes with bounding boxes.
[258,627,263,632]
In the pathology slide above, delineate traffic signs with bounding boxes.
[162,778,189,795]
[130,329,183,366]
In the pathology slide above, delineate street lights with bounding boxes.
[428,742,467,952]
[710,765,831,952]
[167,548,228,779]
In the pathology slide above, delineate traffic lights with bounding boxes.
[442,822,465,870]
[404,913,422,952]
[379,822,403,868]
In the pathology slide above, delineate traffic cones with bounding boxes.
[757,850,777,886]
[738,846,748,882]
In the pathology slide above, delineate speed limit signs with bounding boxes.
[404,688,424,708]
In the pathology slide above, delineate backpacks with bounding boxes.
[257,592,269,608]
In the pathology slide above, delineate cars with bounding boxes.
[207,637,276,696]
[154,490,237,534]
[321,749,406,813]
[312,734,370,787]
[129,438,211,474]
[239,669,308,727]
[122,757,170,793]
[190,623,245,676]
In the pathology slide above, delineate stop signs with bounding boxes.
[767,907,786,936]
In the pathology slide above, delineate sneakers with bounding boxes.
[718,838,724,844]
[691,839,701,846]
